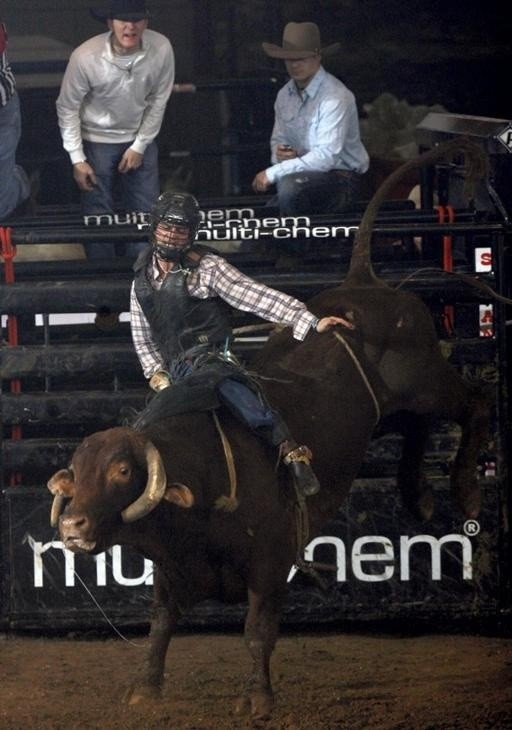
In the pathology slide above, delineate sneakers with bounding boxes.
[93,310,124,333]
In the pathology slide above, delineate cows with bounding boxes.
[44,137,494,716]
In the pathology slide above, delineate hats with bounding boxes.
[89,0,164,23]
[260,20,345,59]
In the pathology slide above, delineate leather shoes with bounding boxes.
[280,441,321,496]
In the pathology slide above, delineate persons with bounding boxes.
[55,0,176,332]
[129,189,357,496]
[250,22,370,272]
[1,24,39,219]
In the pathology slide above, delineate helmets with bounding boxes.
[151,190,201,234]
